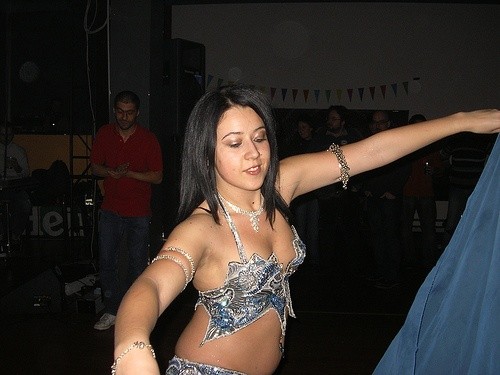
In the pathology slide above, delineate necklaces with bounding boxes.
[213,190,266,233]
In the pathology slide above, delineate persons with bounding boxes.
[291,103,499,292]
[113,82,500,375]
[89,89,163,330]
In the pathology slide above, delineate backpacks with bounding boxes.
[37,159,73,202]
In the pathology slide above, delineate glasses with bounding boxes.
[326,117,346,123]
[370,120,389,125]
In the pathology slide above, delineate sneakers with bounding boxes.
[93,313,117,330]
[0,240,8,258]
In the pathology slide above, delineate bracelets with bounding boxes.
[110,341,156,375]
[157,247,195,281]
[147,255,190,291]
[328,142,351,190]
